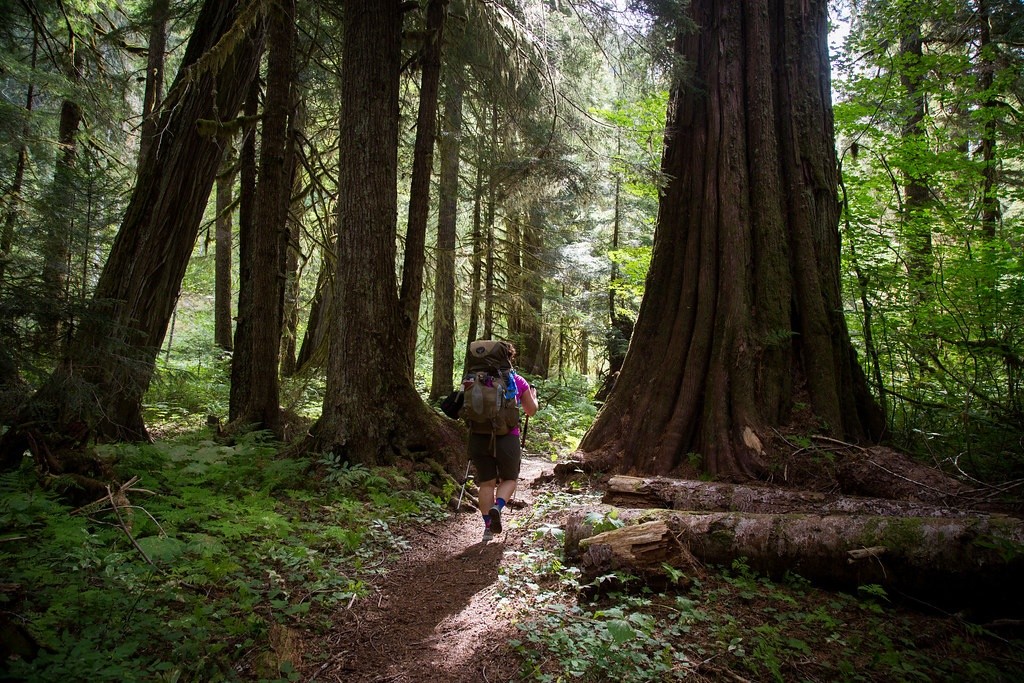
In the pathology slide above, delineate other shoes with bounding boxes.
[488,505,502,534]
[482,528,493,541]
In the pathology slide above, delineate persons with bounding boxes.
[460,342,539,541]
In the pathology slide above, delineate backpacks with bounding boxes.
[461,340,521,438]
